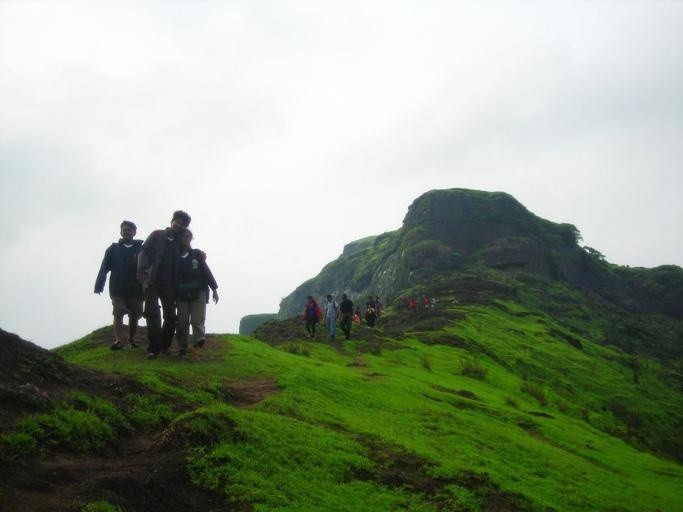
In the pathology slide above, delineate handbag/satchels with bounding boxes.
[178,281,200,301]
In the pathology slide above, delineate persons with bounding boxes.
[138,211,207,361]
[93,220,145,350]
[303,294,442,340]
[173,229,220,356]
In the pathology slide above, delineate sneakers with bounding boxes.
[110,341,124,350]
[128,342,137,349]
[148,347,187,357]
[193,337,206,348]
